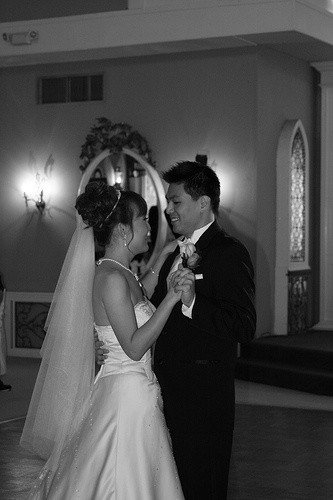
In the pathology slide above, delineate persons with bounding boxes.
[18,178,192,499]
[148,160,256,499]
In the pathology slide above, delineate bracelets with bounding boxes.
[150,268,159,278]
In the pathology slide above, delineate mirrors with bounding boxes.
[76,146,168,281]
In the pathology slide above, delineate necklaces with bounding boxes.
[98,257,142,288]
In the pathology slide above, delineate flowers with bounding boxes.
[177,239,200,270]
